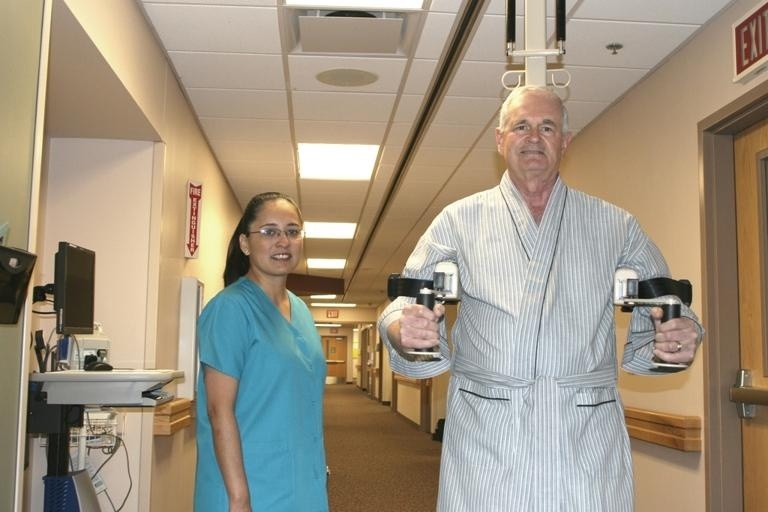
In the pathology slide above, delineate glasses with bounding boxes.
[249,227,307,241]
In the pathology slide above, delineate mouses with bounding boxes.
[84,361,113,371]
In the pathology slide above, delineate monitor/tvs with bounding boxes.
[53,241,96,335]
[0,246,37,324]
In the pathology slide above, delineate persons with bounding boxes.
[374,85,704,512]
[192,191,327,512]
